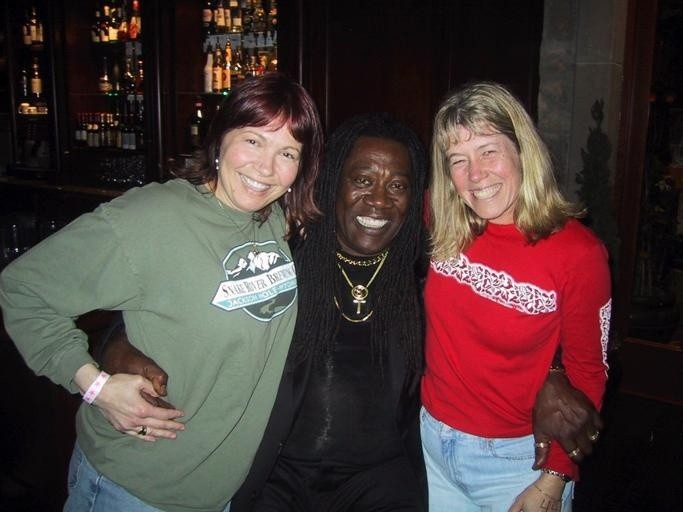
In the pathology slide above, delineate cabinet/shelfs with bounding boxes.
[0,0,303,185]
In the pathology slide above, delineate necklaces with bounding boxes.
[207,181,260,255]
[334,248,389,323]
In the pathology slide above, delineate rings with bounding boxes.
[532,441,550,449]
[568,446,582,459]
[138,425,147,437]
[589,429,600,442]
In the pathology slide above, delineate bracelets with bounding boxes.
[541,467,571,483]
[549,360,566,374]
[81,371,111,405]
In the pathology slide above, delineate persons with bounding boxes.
[417,79,613,512]
[95,105,607,512]
[1,73,325,512]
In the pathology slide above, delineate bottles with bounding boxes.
[73,100,144,151]
[89,1,138,42]
[97,53,141,95]
[199,1,277,96]
[19,56,41,100]
[21,7,42,46]
[187,101,206,150]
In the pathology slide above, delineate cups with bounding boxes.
[16,103,29,114]
[25,106,36,114]
